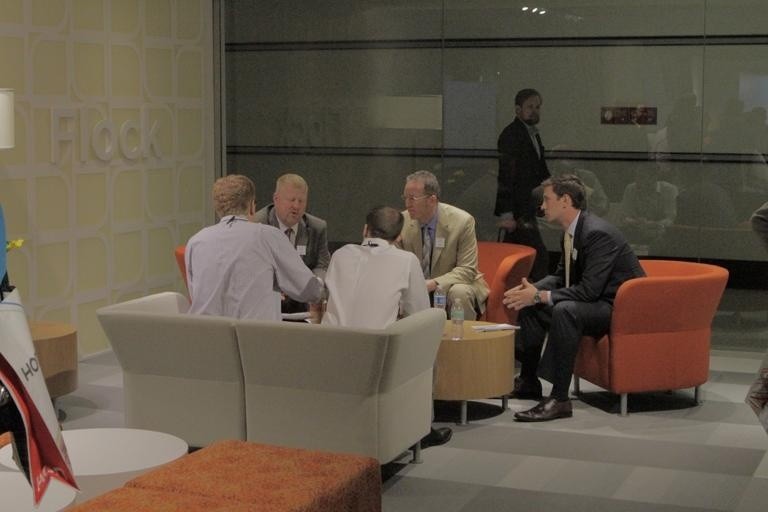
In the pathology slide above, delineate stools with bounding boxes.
[59,487,272,511]
[0,469,80,510]
[0,422,189,501]
[125,436,387,510]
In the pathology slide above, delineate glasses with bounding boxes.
[401,194,429,202]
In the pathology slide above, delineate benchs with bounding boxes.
[73,442,382,512]
[96,291,447,467]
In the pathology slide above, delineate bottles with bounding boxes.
[450,298,465,342]
[432,285,447,310]
[309,295,327,325]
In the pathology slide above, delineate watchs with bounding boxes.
[534,290,542,304]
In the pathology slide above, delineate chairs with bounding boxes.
[171,240,192,287]
[561,258,731,415]
[472,239,538,332]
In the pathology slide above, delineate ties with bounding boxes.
[563,231,572,290]
[421,226,432,279]
[284,228,293,241]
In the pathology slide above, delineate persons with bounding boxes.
[493,88,551,281]
[251,174,331,322]
[186,175,324,322]
[745,199,768,412]
[618,163,678,244]
[320,206,452,451]
[750,202,768,252]
[555,160,609,218]
[676,170,738,230]
[502,175,648,422]
[392,170,490,320]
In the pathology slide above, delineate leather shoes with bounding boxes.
[489,375,543,401]
[515,398,573,421]
[407,427,452,450]
